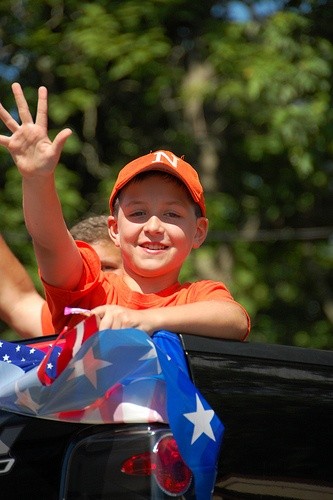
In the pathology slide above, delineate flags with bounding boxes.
[0,306,224,500]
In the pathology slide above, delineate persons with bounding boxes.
[0,83,251,341]
[0,215,124,338]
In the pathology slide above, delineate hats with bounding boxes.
[108,149,206,217]
